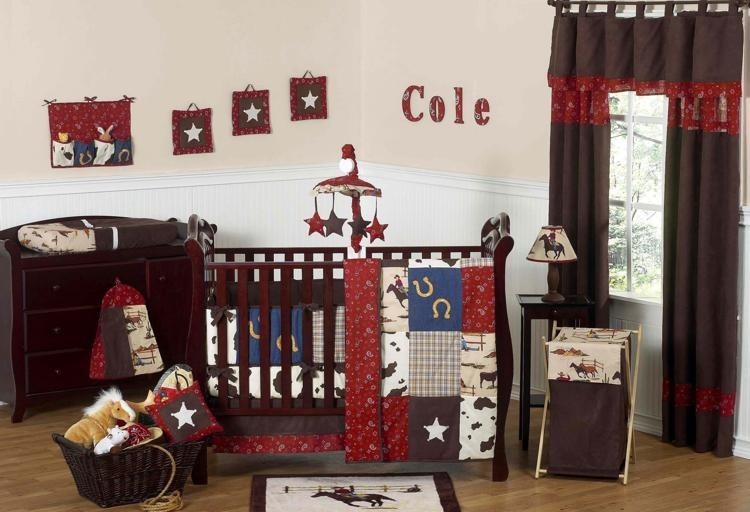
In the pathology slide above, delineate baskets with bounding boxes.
[52,431,204,508]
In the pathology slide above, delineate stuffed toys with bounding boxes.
[64,388,136,450]
[94,425,130,455]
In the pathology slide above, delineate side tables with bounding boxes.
[518,293,596,451]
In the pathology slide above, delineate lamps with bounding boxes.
[525,225,577,302]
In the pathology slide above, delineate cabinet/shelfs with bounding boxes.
[0,215,217,423]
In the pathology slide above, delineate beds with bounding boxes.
[184,211,514,485]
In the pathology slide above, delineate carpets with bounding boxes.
[250,470,462,512]
